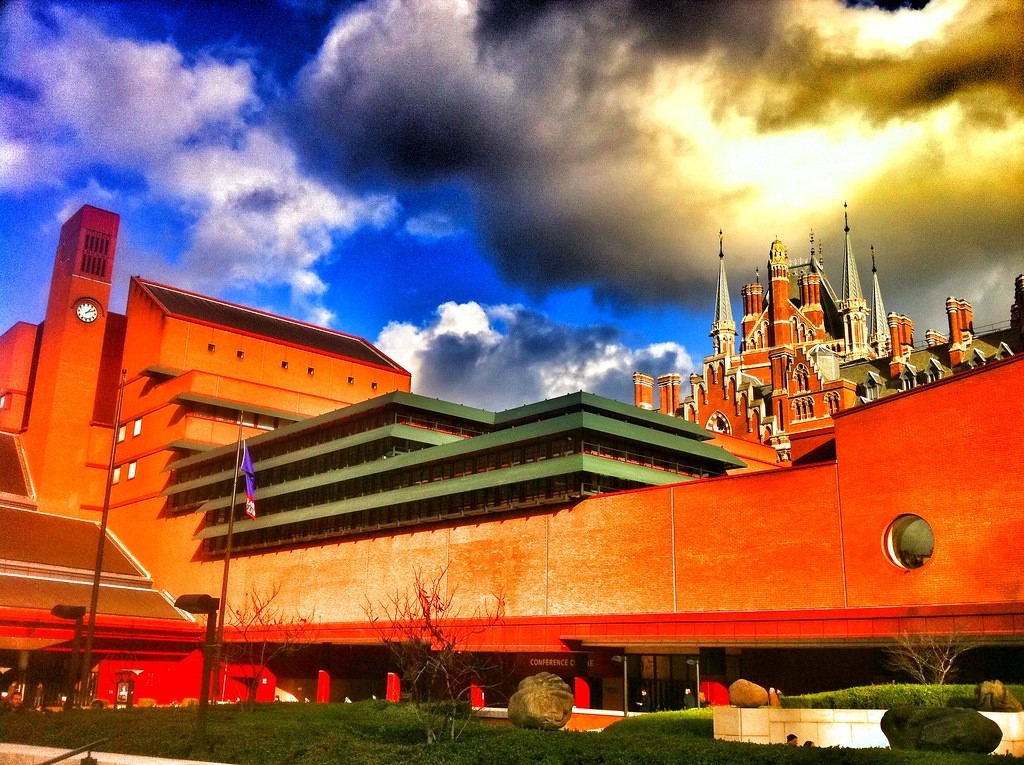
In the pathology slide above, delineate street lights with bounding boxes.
[686,659,700,709]
[49,605,88,711]
[611,656,628,718]
[174,593,219,732]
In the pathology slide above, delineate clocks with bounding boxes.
[77,303,98,323]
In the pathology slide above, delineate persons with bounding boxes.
[636,688,650,712]
[787,734,798,745]
[803,741,815,747]
[683,687,695,708]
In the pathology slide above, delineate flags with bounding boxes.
[241,440,256,520]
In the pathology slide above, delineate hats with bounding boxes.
[787,734,797,742]
